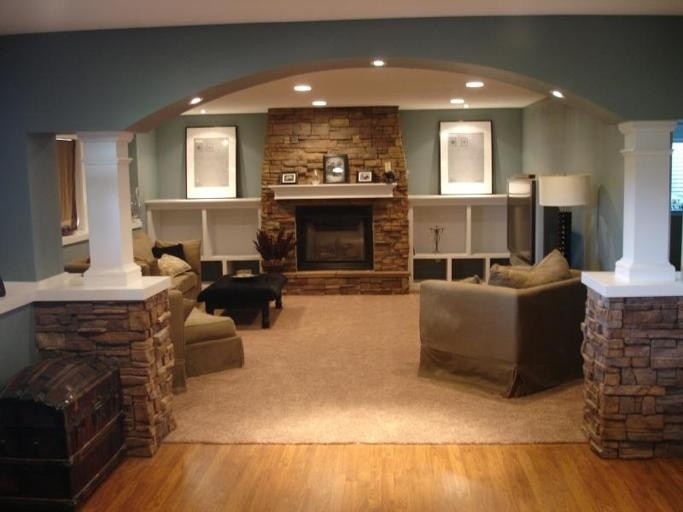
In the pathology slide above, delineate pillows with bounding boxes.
[182,297,198,324]
[462,249,571,288]
[131,231,191,278]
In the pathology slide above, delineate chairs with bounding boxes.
[416,267,587,399]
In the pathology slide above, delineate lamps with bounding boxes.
[537,174,591,269]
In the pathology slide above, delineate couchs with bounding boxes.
[64,227,245,392]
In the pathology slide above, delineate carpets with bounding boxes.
[154,281,590,443]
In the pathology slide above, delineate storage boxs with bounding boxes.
[0,354,130,511]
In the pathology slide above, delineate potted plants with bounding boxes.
[251,226,297,272]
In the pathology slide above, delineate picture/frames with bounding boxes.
[323,154,348,184]
[183,123,238,200]
[436,118,494,197]
[355,170,374,183]
[279,171,297,185]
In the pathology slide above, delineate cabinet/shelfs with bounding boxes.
[140,198,264,276]
[408,193,509,290]
